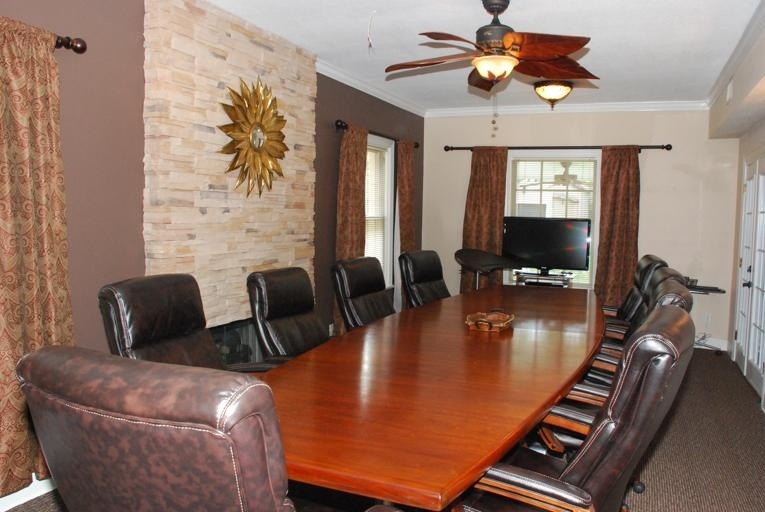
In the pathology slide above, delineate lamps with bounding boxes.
[532,81,572,111]
[471,55,520,81]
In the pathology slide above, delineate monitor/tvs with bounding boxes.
[502,216,591,277]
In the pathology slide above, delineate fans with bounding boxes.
[384,0,600,91]
[520,161,593,191]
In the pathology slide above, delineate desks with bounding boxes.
[263,284,606,511]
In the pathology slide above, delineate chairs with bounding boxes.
[604,267,694,345]
[399,250,451,307]
[446,304,694,511]
[98,273,278,373]
[331,258,397,331]
[605,255,670,326]
[248,266,334,362]
[15,343,401,511]
[577,278,694,392]
